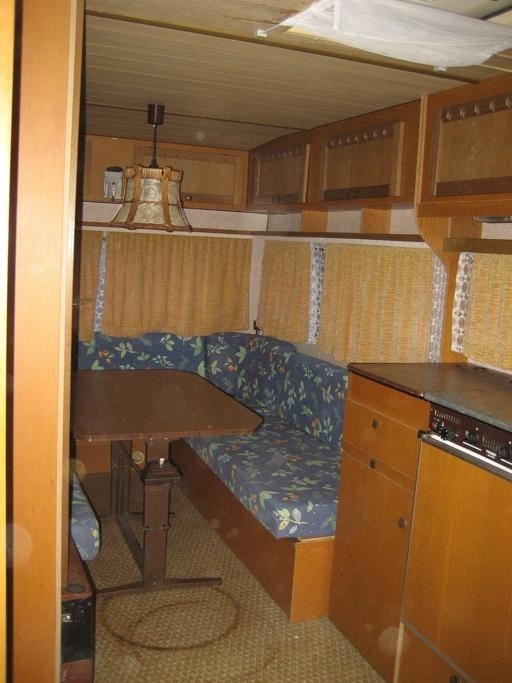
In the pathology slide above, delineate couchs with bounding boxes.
[66,329,348,623]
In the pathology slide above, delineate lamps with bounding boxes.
[110,103,192,233]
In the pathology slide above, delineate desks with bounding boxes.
[70,369,264,598]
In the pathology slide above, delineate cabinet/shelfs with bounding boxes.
[84,134,249,212]
[393,439,512,682]
[247,74,425,217]
[415,71,512,218]
[327,371,430,682]
